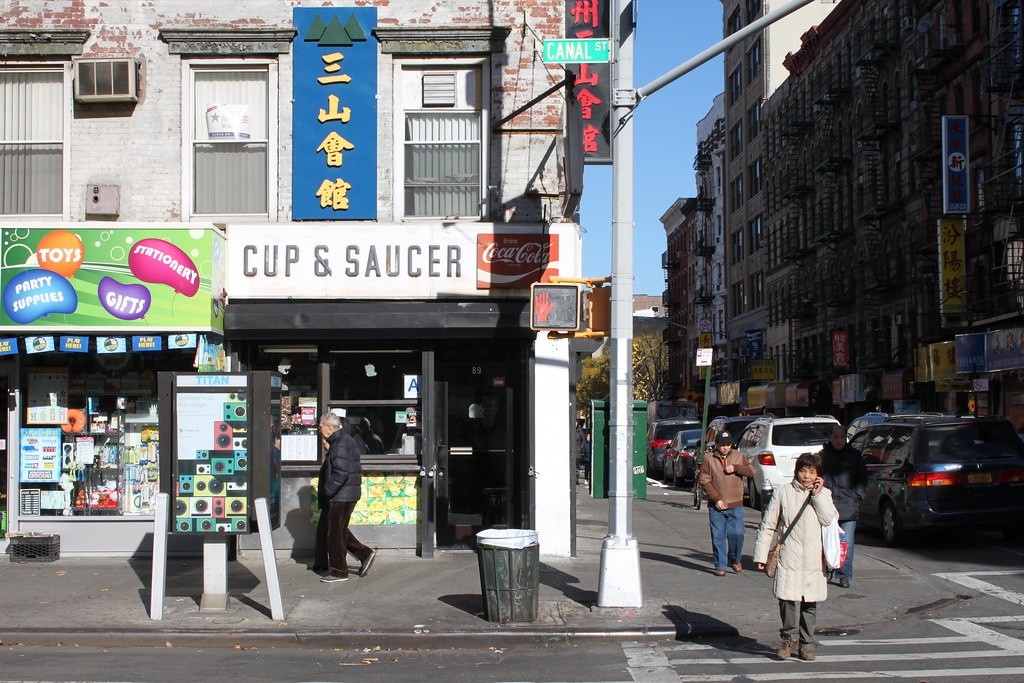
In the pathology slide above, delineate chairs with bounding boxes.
[940,432,975,455]
[779,430,800,446]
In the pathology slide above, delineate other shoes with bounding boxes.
[313,563,329,571]
[840,577,849,588]
[359,552,376,575]
[826,571,832,580]
[321,570,349,583]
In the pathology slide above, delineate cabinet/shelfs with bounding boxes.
[57,397,120,515]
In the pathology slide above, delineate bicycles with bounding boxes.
[690,451,702,510]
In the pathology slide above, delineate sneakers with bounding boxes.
[777,639,799,659]
[797,648,815,661]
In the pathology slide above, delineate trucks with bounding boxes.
[647,400,699,427]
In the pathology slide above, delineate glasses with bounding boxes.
[318,425,326,430]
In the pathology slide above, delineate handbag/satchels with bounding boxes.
[765,551,778,577]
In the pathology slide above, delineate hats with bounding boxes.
[715,430,734,444]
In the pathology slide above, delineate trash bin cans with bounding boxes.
[476,528,540,623]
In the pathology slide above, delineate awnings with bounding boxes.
[881,368,922,400]
[747,378,826,409]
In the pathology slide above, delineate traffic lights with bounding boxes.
[530,282,581,332]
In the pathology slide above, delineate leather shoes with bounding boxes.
[717,570,725,575]
[732,562,741,572]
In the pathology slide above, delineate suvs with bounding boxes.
[706,412,780,451]
[844,410,945,451]
[732,414,850,510]
[645,417,702,480]
[848,414,1024,550]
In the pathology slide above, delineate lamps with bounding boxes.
[258,344,317,353]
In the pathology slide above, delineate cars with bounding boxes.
[662,428,707,487]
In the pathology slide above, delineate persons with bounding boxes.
[752,453,845,662]
[305,411,376,584]
[700,430,757,576]
[350,417,386,455]
[580,432,590,485]
[818,425,869,588]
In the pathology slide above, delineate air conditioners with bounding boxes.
[859,176,865,184]
[950,33,961,46]
[813,105,826,113]
[902,17,914,32]
[73,59,139,103]
[865,52,879,60]
[894,152,901,161]
[896,313,905,325]
[916,57,925,69]
[910,100,917,111]
